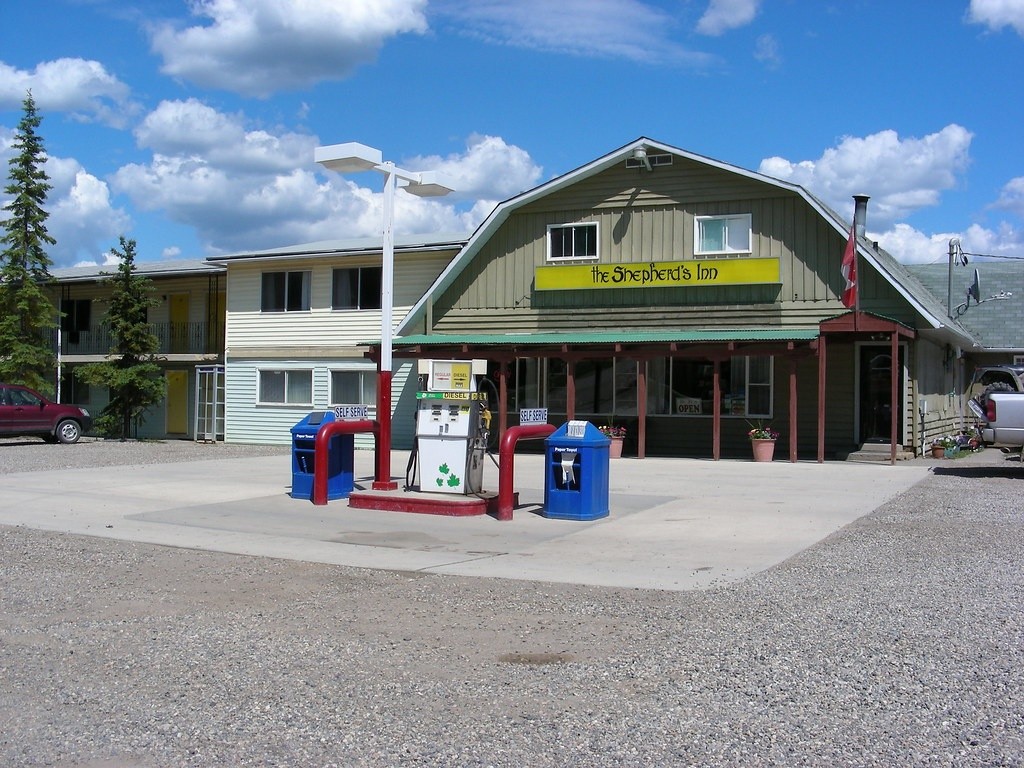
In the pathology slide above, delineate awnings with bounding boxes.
[356,330,819,345]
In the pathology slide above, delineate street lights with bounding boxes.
[313,142,457,491]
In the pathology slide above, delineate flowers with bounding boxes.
[599,412,627,441]
[932,427,980,447]
[745,416,780,441]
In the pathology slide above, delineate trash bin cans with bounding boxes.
[288,411,354,501]
[542,421,611,520]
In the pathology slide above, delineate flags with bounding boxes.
[840,219,859,310]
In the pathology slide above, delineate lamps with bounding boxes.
[633,145,652,171]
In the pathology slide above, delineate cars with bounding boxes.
[0,384,94,445]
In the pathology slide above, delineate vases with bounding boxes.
[957,434,970,444]
[609,437,625,459]
[931,446,945,457]
[751,439,775,462]
[971,438,979,446]
[944,447,953,457]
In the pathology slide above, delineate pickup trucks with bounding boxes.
[982,391,1024,452]
